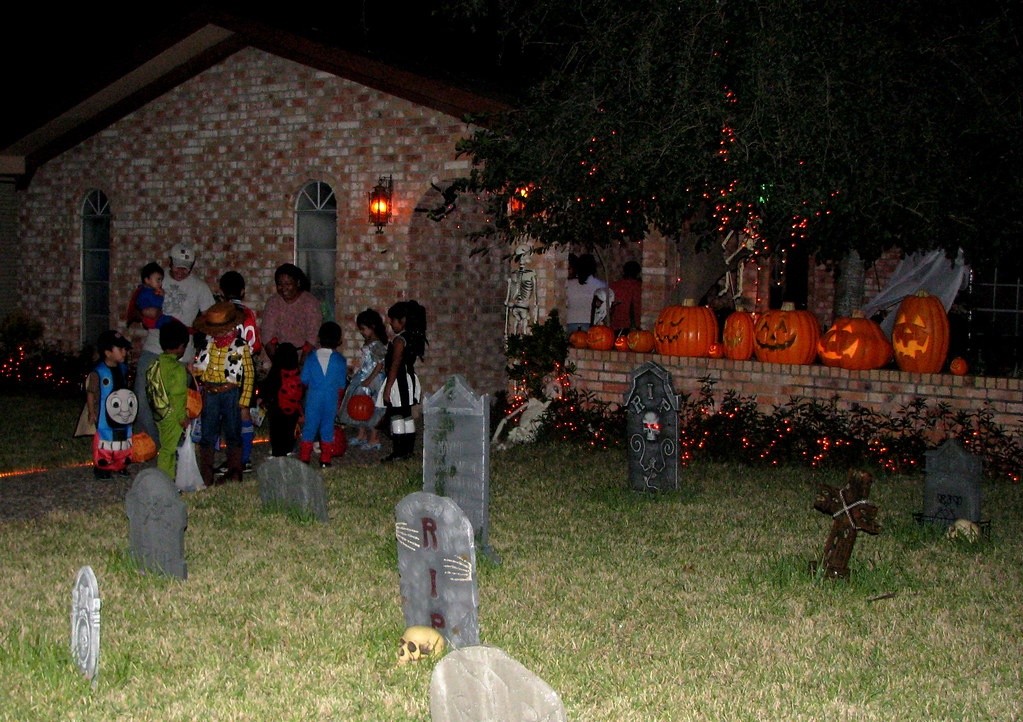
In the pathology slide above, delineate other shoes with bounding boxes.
[241,460,252,472]
[93,465,112,481]
[359,443,381,451]
[213,464,227,474]
[348,437,368,446]
[113,468,133,477]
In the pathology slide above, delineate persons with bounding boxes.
[74,328,157,482]
[127,242,323,489]
[301,321,348,468]
[490,380,564,450]
[561,250,643,334]
[395,623,445,665]
[946,518,980,545]
[334,308,390,451]
[501,242,539,394]
[373,300,429,465]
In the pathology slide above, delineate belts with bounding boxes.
[203,381,236,393]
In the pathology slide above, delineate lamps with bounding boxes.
[367,175,394,234]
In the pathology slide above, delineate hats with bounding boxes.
[168,244,196,270]
[196,301,243,334]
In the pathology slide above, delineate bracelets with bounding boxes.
[366,378,371,385]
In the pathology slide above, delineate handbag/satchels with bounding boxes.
[184,368,206,419]
[130,431,158,461]
[174,424,209,493]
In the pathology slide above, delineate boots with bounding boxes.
[403,418,417,462]
[380,418,405,464]
[200,447,216,487]
[217,447,243,485]
[299,441,314,463]
[319,441,334,467]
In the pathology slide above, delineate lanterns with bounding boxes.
[627,329,655,353]
[752,300,824,366]
[893,289,950,375]
[652,298,718,357]
[569,330,587,348]
[708,341,724,359]
[949,355,967,376]
[615,334,630,353]
[723,303,754,363]
[586,320,614,351]
[347,384,376,422]
[816,309,892,371]
